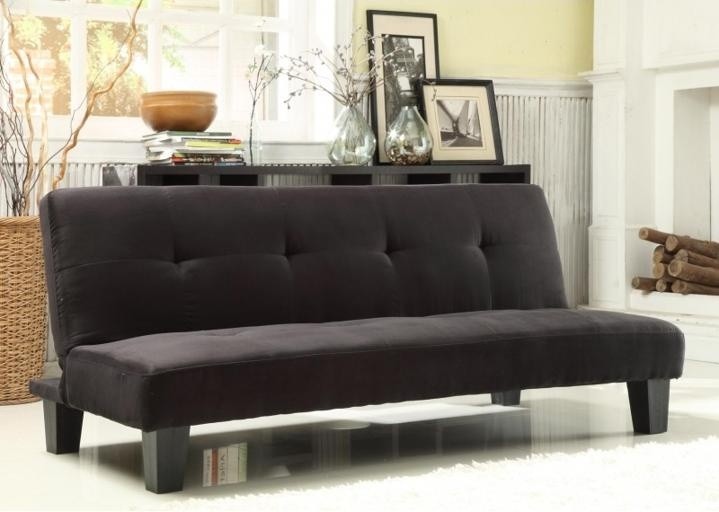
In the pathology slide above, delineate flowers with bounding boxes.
[275,25,404,107]
[243,42,283,165]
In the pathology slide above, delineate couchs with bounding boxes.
[30,181,686,495]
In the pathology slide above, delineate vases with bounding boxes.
[325,106,379,168]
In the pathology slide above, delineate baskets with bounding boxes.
[1,215,48,406]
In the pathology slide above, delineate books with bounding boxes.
[141,130,246,168]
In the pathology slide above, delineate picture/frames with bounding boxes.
[367,8,506,165]
